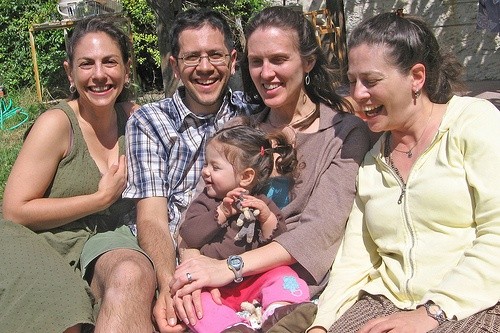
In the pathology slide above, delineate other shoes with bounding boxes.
[221,324,259,333]
[259,302,318,333]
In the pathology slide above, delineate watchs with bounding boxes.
[424,299,448,325]
[227,255,244,284]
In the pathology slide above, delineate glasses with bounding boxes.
[175,51,231,66]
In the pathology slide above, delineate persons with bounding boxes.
[122,8,265,333]
[169,5,367,333]
[304,7,500,333]
[0,13,159,333]
[177,123,317,333]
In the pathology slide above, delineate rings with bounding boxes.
[187,273,191,282]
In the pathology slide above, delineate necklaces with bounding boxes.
[391,103,435,158]
[268,107,316,128]
